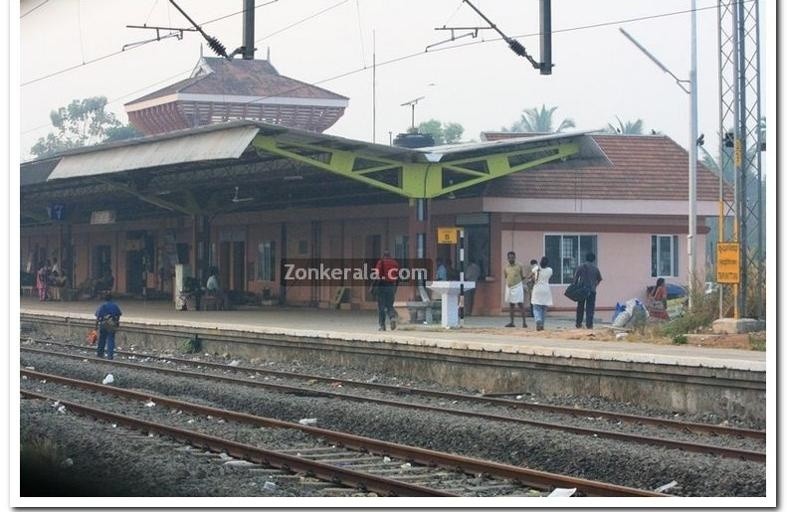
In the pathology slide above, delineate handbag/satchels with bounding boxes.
[86,329,97,345]
[565,283,591,302]
[650,301,665,312]
[369,278,394,296]
[102,314,120,334]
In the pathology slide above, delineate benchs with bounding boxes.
[21,285,77,302]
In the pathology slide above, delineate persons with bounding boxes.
[645,277,672,321]
[94,294,123,362]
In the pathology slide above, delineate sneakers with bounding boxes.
[379,318,396,331]
[576,324,593,329]
[536,320,543,331]
